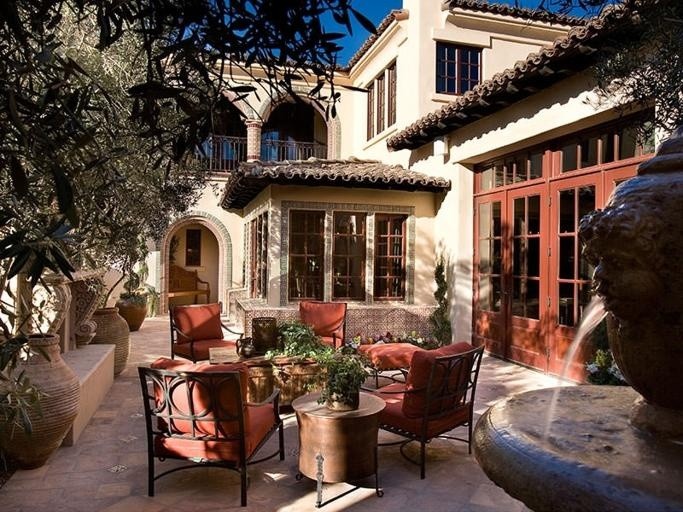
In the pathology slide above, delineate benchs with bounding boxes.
[59,342,117,448]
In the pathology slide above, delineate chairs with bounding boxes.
[169,264,210,309]
[137,356,284,507]
[167,301,245,364]
[297,301,347,352]
[358,339,485,478]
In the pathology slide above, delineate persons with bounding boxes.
[576,206,677,328]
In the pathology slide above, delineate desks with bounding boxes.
[291,390,386,507]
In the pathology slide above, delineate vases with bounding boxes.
[89,307,130,377]
[0,333,82,471]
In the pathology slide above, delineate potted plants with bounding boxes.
[312,350,371,411]
[114,292,150,332]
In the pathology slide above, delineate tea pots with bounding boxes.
[234,337,257,358]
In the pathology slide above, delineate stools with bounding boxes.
[357,343,427,393]
